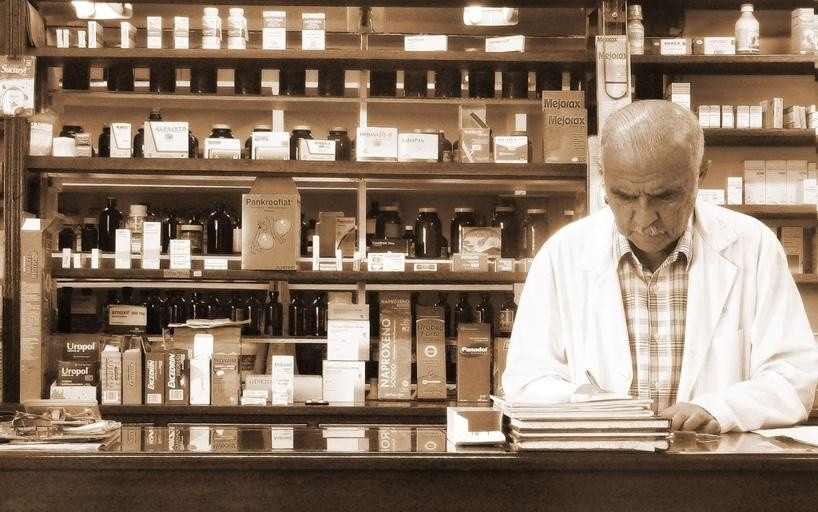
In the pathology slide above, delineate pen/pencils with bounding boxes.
[585,371,602,389]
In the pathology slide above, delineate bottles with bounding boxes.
[59,105,533,163]
[734,4,761,56]
[226,7,249,51]
[202,7,224,48]
[59,288,516,341]
[60,194,574,271]
[626,4,644,56]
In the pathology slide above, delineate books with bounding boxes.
[490,395,673,452]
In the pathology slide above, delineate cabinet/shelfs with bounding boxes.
[25,0,816,423]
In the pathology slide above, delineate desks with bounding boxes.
[0,425,816,512]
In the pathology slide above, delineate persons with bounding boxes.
[503,99,818,435]
